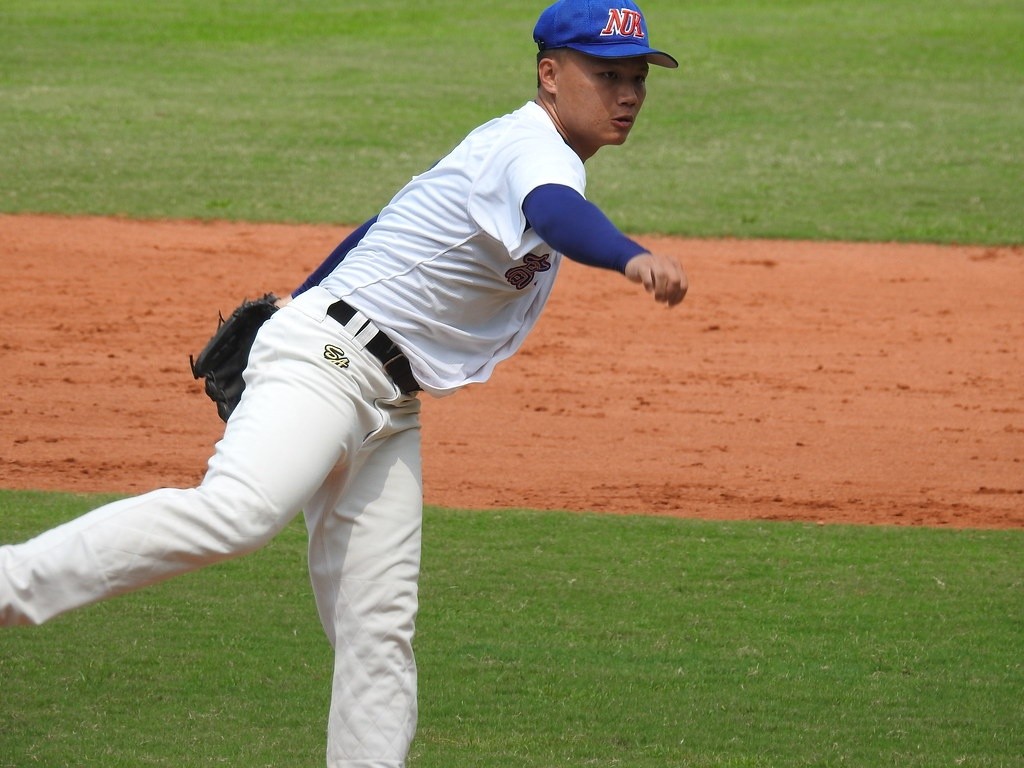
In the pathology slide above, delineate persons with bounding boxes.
[0,0,687,767]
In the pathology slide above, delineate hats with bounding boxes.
[533,0,679,69]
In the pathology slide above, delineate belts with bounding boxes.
[327,300,424,395]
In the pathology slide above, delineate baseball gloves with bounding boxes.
[188,289,281,424]
